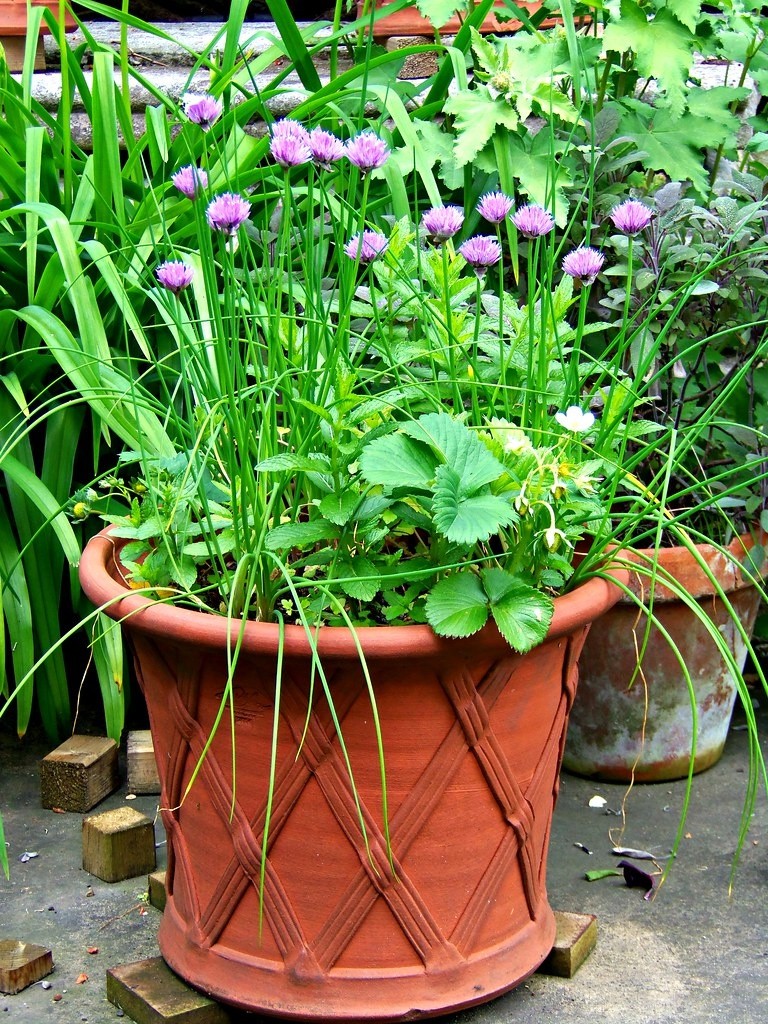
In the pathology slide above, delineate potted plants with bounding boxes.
[561,215,768,783]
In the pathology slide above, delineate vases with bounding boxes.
[82,513,627,1024]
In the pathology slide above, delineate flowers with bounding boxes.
[0,100,767,946]
